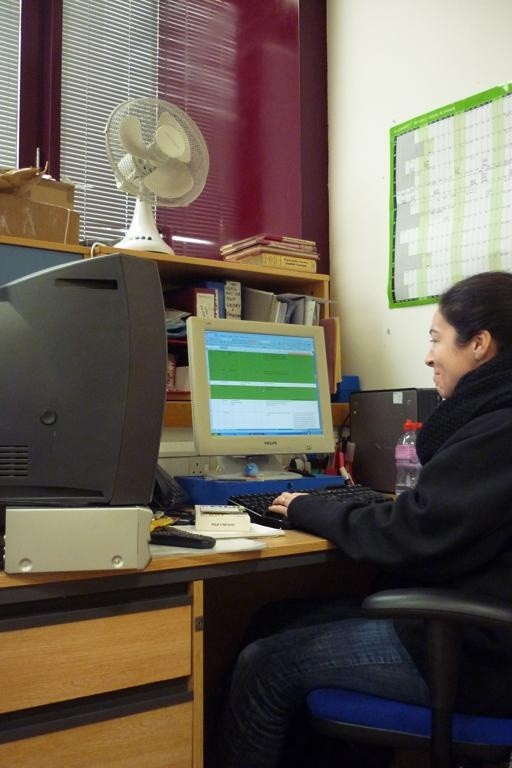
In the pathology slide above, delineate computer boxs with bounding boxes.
[3,509,152,573]
[348,390,441,494]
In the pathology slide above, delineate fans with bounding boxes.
[101,96,212,255]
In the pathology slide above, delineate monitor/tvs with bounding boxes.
[186,313,338,482]
[0,254,167,507]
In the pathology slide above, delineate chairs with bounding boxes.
[305,583,510,767]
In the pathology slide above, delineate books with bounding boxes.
[150,536,270,559]
[162,278,344,397]
[218,231,320,273]
[168,521,287,539]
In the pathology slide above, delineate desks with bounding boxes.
[0,488,391,766]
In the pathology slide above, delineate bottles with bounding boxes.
[392,418,422,496]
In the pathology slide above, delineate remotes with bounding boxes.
[151,528,216,548]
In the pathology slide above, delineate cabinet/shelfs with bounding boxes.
[92,248,345,425]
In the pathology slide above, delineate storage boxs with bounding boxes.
[0,166,82,243]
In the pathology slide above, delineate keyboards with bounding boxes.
[229,484,392,529]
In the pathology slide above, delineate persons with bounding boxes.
[218,267,512,767]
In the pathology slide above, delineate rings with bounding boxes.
[281,495,288,504]
